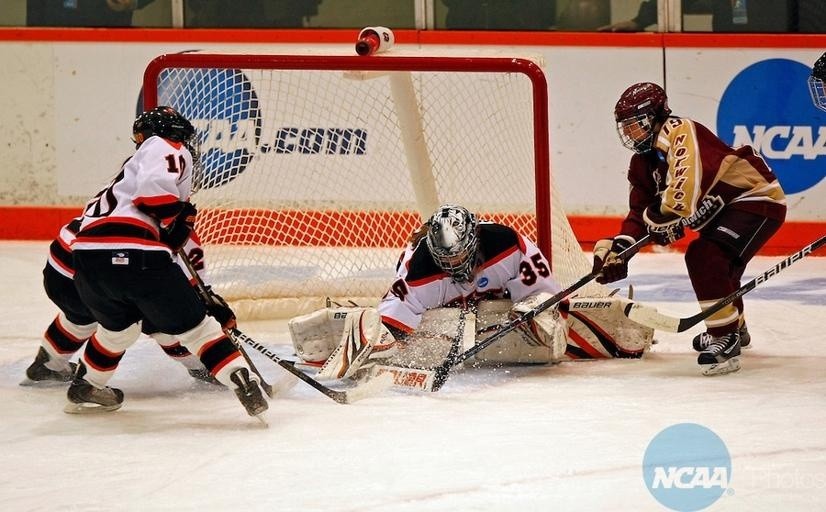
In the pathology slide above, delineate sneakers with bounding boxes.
[693,320,750,351]
[698,326,741,364]
[230,367,268,417]
[26,345,78,382]
[187,367,223,385]
[67,357,124,406]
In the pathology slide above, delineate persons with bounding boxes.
[63,106,272,419]
[290,204,659,395]
[593,1,826,33]
[182,0,321,27]
[591,81,788,366]
[27,0,157,27]
[442,0,556,31]
[22,141,229,387]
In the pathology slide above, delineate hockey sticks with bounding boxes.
[229,327,393,404]
[624,235,825,332]
[178,247,300,398]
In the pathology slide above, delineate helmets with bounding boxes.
[614,82,672,154]
[426,205,480,283]
[133,107,204,198]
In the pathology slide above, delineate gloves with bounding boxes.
[160,201,197,250]
[643,202,685,246]
[206,304,237,332]
[592,235,639,284]
